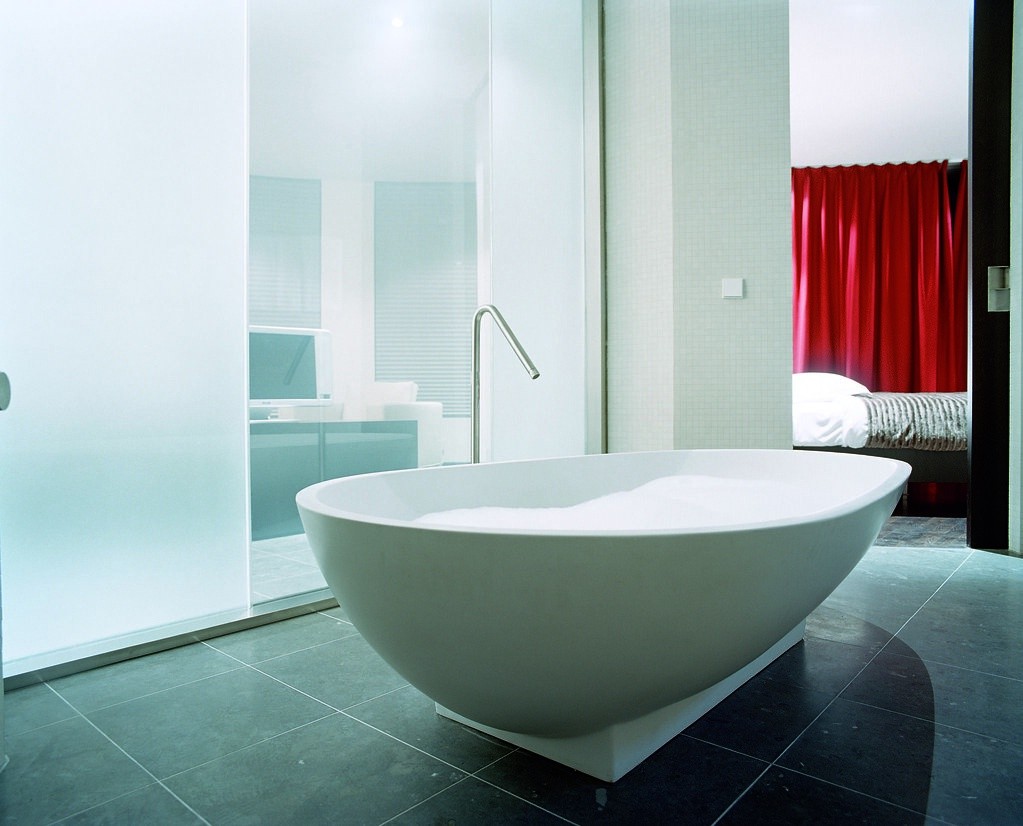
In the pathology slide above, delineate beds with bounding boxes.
[790,390,969,454]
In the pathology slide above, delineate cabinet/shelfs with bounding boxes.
[248,401,447,608]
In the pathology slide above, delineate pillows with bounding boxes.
[791,370,875,401]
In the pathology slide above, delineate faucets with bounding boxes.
[467,304,542,465]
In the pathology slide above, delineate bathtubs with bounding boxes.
[292,445,915,744]
